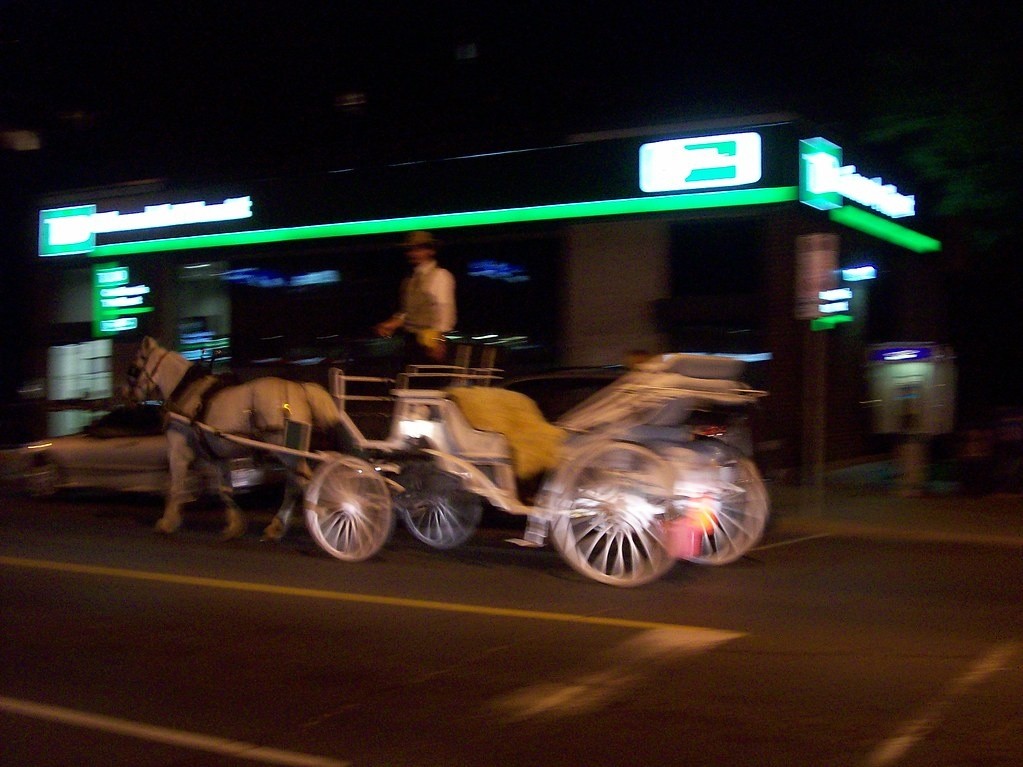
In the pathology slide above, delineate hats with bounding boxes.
[397,230,443,249]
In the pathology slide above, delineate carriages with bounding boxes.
[112,337,771,589]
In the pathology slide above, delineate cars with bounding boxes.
[17,407,289,506]
[503,366,773,487]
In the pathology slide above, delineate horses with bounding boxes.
[115,335,339,544]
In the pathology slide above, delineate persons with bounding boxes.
[375,228,458,390]
